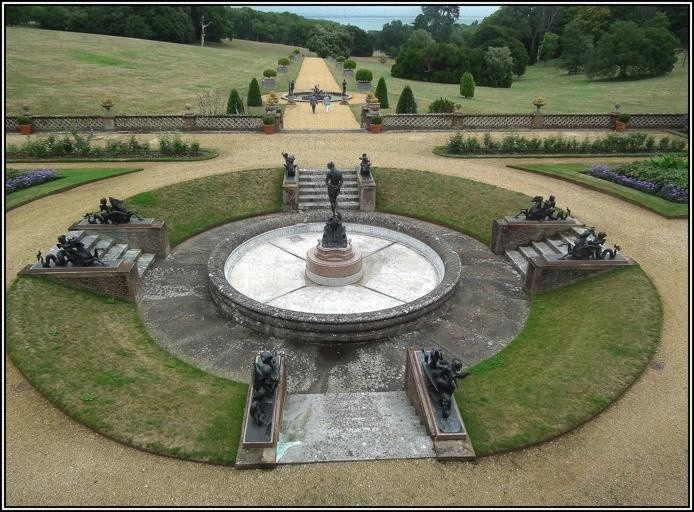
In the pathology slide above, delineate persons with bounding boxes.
[358,153,373,168]
[324,160,344,218]
[309,94,319,115]
[342,79,347,94]
[291,80,295,95]
[540,194,556,217]
[98,197,107,212]
[586,228,608,253]
[251,349,276,397]
[54,234,77,258]
[322,95,331,112]
[281,150,296,174]
[431,351,470,392]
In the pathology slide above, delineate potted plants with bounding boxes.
[98,96,115,112]
[532,95,546,108]
[370,117,381,133]
[16,114,33,135]
[615,112,630,131]
[262,115,274,133]
